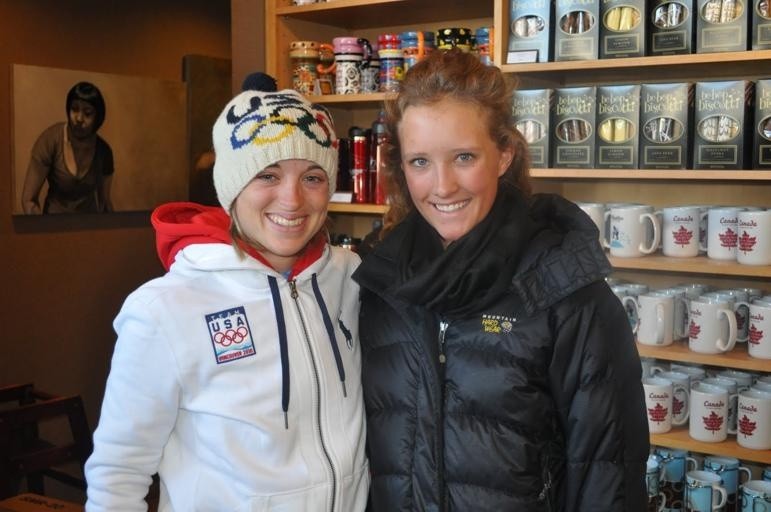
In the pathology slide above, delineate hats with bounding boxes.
[212,73,340,215]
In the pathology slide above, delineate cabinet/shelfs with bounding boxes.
[263,0,771,512]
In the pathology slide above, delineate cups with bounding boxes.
[761,466,771,483]
[645,460,666,497]
[655,447,698,509]
[742,480,771,512]
[602,276,771,359]
[703,454,752,512]
[574,202,771,265]
[641,358,771,451]
[684,470,728,512]
[647,492,667,512]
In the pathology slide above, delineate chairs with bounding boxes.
[0,380,93,502]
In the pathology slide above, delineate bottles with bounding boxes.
[336,237,361,250]
[289,27,494,100]
[335,111,394,205]
[360,220,385,259]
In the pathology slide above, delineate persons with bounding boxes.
[352,48,650,512]
[194,72,277,208]
[21,82,115,215]
[82,89,373,512]
